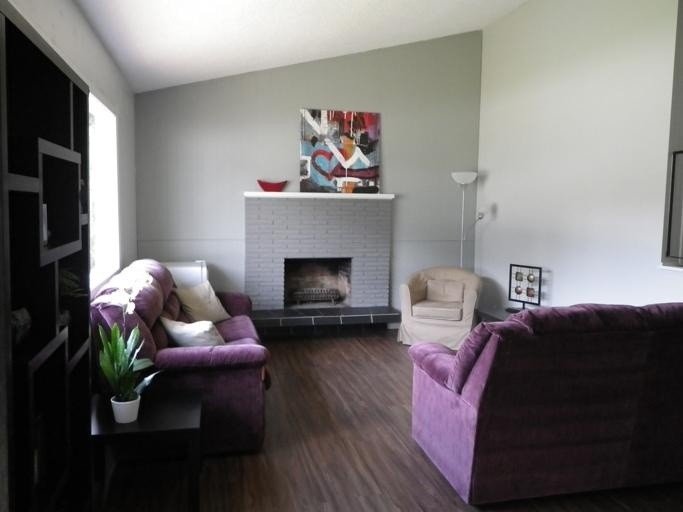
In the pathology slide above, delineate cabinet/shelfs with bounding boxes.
[0,0,111,512]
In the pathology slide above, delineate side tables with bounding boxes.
[90,389,203,510]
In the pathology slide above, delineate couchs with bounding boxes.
[90,258,272,464]
[408,303,683,506]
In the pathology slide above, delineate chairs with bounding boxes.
[396,266,483,351]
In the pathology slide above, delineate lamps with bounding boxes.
[451,171,484,268]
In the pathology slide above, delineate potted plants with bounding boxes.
[97,321,169,424]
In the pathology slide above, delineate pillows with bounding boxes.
[426,280,465,303]
[159,315,226,347]
[170,279,232,324]
